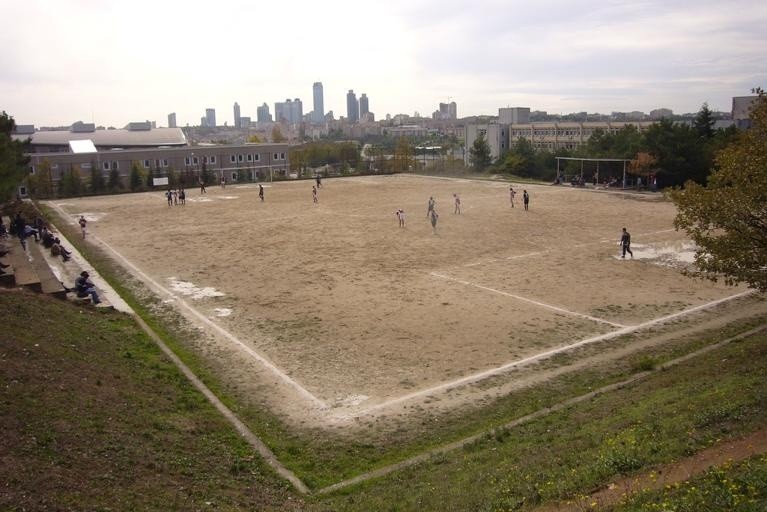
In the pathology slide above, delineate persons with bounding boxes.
[426,196,439,235]
[79,215,87,239]
[555,169,643,189]
[258,184,264,200]
[509,187,530,211]
[453,193,461,216]
[396,209,406,227]
[0,210,72,278]
[165,187,185,206]
[75,271,102,304]
[620,227,633,258]
[312,175,323,202]
